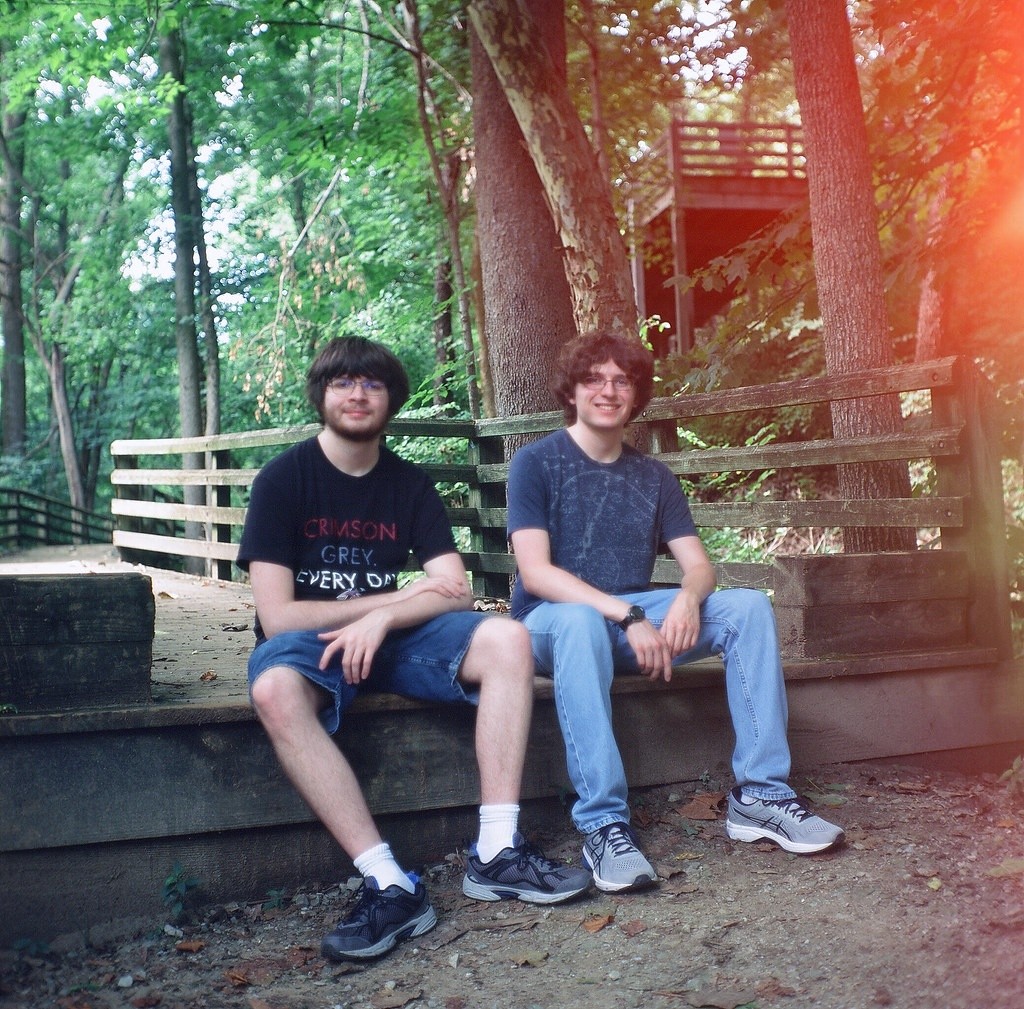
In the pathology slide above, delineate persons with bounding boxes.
[236,337,596,961]
[506,337,846,894]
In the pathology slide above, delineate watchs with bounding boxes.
[619,605,646,631]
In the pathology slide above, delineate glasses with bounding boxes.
[576,376,638,391]
[326,377,387,396]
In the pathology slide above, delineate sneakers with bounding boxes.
[463,831,596,905]
[726,786,846,856]
[581,822,659,895]
[321,870,438,962]
